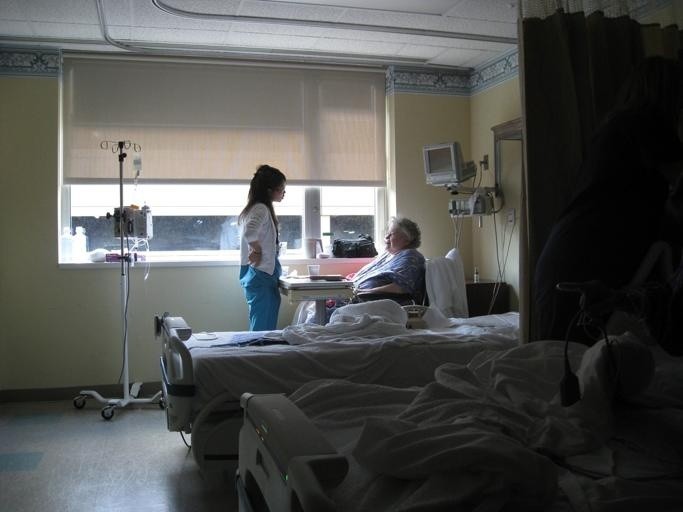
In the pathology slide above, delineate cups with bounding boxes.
[307,264,321,278]
[281,266,289,279]
[279,241,288,257]
[303,238,323,258]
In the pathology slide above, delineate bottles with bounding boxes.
[473,265,480,283]
[58,226,87,264]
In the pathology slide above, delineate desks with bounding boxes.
[278,275,353,325]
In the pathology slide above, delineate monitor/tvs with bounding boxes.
[422,141,464,185]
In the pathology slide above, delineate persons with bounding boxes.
[303,216,425,326]
[238,164,286,330]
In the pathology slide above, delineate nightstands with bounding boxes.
[466,279,509,317]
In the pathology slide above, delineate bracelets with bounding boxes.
[251,249,262,254]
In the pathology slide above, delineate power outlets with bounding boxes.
[506,208,515,226]
[482,154,489,169]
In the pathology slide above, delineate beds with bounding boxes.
[154,312,519,467]
[235,338,682,511]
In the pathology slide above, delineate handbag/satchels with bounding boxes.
[332,233,378,258]
